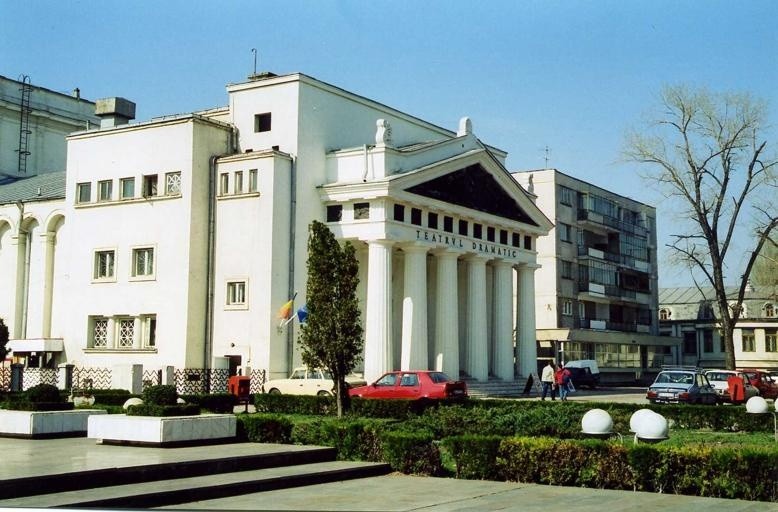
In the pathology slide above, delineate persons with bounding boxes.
[554,364,571,402]
[540,361,556,401]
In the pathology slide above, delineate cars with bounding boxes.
[261,362,367,395]
[552,354,778,410]
[346,369,468,398]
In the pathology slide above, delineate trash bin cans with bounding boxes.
[228,376,250,401]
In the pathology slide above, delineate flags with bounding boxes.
[275,295,297,320]
[297,304,308,322]
